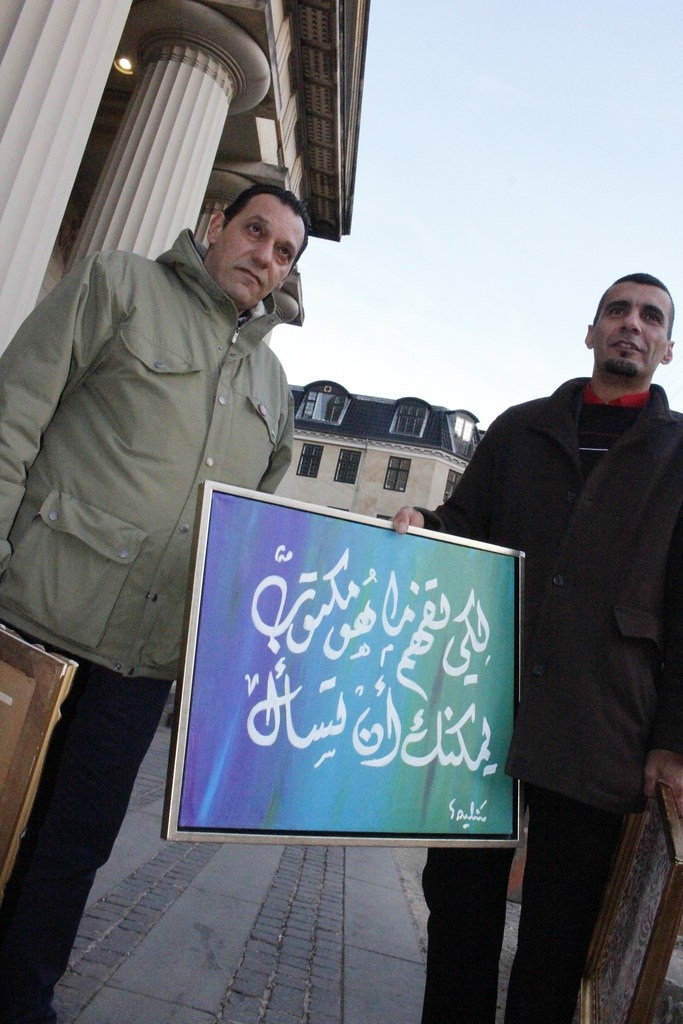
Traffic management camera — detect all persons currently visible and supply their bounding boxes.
[393,272,683,1024]
[0,183,311,1024]
